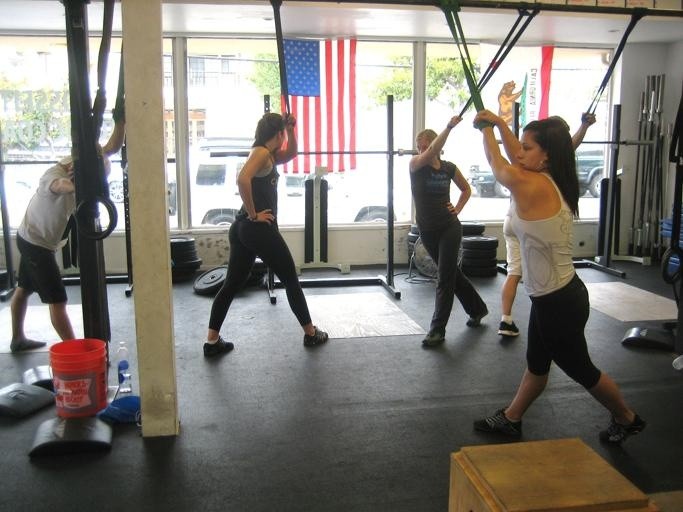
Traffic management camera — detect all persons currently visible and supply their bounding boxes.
[9,99,126,351]
[498,112,595,337]
[407,116,489,347]
[473,106,646,447]
[203,112,329,357]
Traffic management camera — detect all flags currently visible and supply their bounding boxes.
[277,37,357,177]
[479,42,553,173]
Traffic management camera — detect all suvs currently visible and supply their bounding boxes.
[466,131,607,197]
[187,131,400,229]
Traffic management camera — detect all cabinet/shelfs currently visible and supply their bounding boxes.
[448,438,659,512]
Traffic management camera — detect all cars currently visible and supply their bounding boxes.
[2,151,177,234]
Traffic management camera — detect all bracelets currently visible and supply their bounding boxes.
[478,120,492,130]
[447,123,453,129]
[250,212,258,220]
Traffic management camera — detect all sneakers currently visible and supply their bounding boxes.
[423,329,445,345]
[474,410,521,435]
[498,321,520,336]
[304,326,327,346]
[204,336,233,355]
[600,413,645,445]
[467,304,488,326]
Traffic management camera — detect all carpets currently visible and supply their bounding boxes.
[0,304,84,353]
[583,282,679,322]
[304,292,428,339]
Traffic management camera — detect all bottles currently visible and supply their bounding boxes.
[116,340,133,393]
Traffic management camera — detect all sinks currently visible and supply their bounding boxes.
[48,339,110,418]
